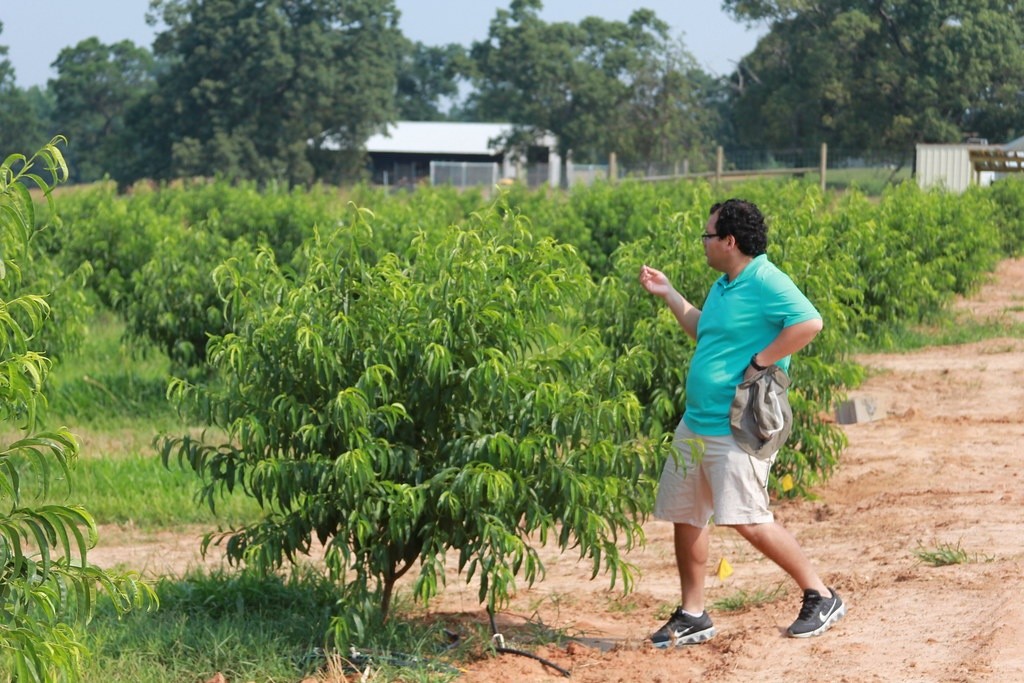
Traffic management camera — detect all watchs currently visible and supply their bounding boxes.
[751,353,767,371]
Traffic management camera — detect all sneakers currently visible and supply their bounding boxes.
[787,587,846,638]
[650,606,717,648]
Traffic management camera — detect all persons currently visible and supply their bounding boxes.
[640,198,846,648]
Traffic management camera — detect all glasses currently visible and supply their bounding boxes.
[701,231,718,243]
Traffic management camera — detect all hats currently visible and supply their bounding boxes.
[730,365,793,460]
